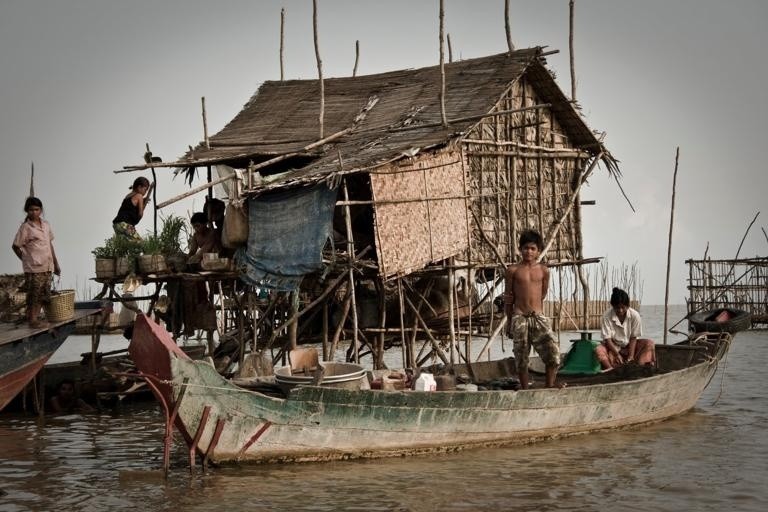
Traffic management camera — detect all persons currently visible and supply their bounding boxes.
[501,229,561,390]
[595,286,656,374]
[112,176,152,245]
[195,198,239,260]
[49,377,95,415]
[186,212,220,256]
[12,195,61,332]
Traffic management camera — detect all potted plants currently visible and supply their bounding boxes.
[91,209,193,278]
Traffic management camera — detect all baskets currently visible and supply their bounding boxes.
[41,289,75,322]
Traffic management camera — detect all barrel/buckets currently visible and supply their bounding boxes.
[277,363,366,392]
[414,369,437,392]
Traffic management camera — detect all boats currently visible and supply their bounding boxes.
[0,303,104,413]
[122,309,749,467]
